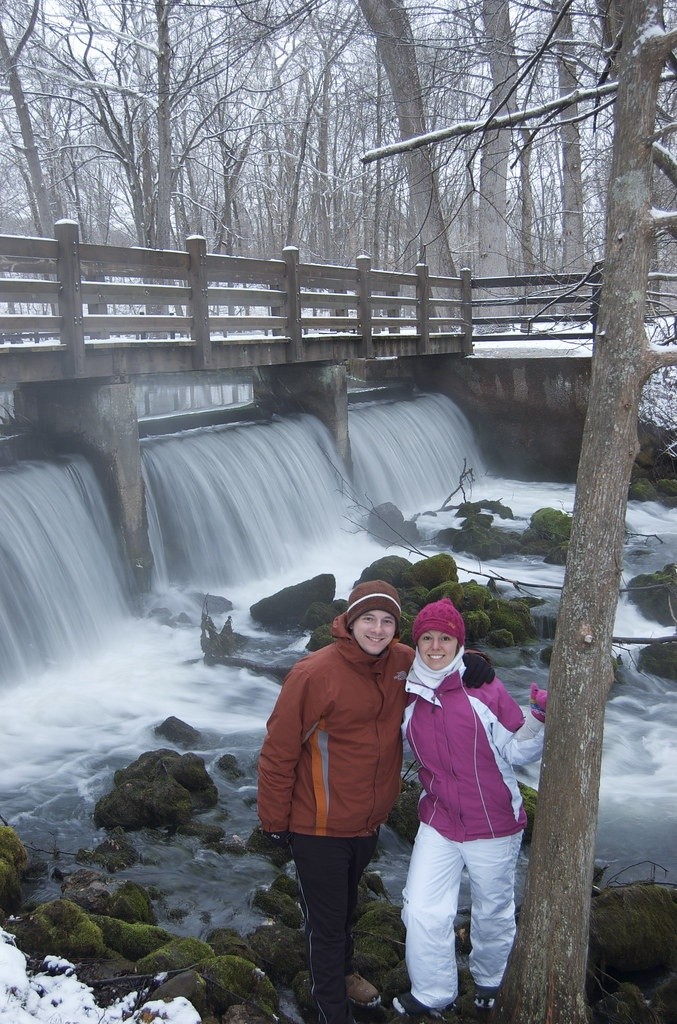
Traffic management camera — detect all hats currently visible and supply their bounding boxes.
[345,579,401,633]
[413,598,465,645]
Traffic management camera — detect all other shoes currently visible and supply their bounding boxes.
[391,993,453,1016]
[472,986,498,1010]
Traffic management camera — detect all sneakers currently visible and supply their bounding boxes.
[344,972,381,1007]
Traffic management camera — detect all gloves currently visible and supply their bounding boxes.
[463,654,495,689]
[267,831,290,845]
[529,683,551,723]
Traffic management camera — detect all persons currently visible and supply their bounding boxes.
[393,599,548,1015]
[258,579,496,1024]
